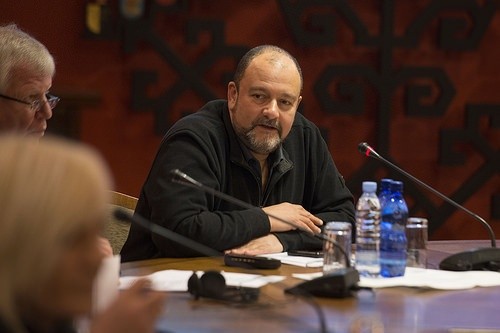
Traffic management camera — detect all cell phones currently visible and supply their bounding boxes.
[225,252,281,269]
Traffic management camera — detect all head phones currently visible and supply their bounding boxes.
[187,270,260,302]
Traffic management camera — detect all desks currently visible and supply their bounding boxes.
[88,240,500,333]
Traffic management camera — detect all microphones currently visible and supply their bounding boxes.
[113,206,328,333]
[357,141,500,271]
[168,169,359,297]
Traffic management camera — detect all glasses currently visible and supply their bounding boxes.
[0,91,61,113]
[188,269,261,305]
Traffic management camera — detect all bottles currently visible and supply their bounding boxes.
[353,181,382,278]
[379,181,409,278]
[377,178,394,212]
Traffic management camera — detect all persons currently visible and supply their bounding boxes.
[0,25,114,257]
[0,137,168,333]
[119,45,357,264]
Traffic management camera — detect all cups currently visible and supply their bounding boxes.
[323,222,352,273]
[406,217,429,271]
